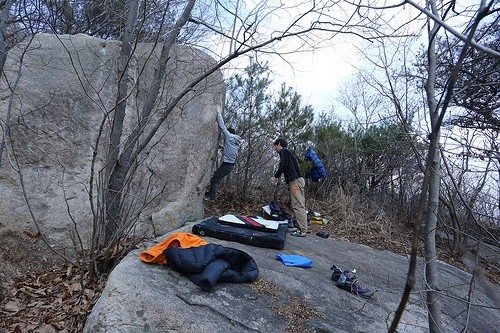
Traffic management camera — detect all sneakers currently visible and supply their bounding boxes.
[331,268,374,299]
[290,229,306,237]
[203,197,215,202]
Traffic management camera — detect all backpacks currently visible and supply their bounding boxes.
[262,201,287,221]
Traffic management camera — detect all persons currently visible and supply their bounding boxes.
[204,107,241,200]
[271,137,308,236]
